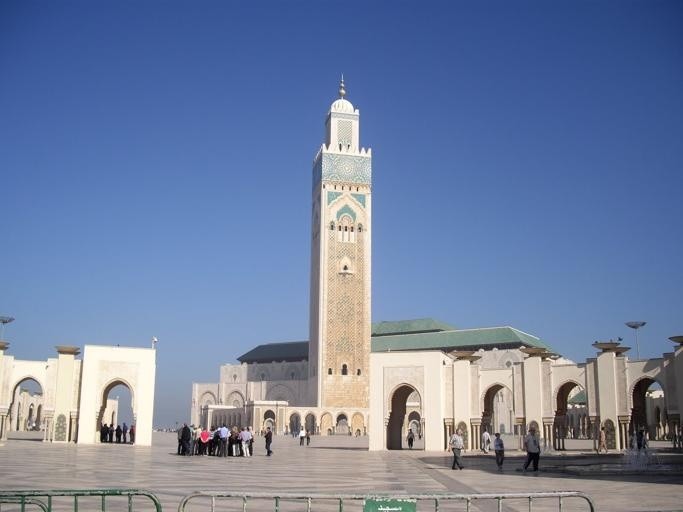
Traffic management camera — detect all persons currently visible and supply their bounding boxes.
[406,429,415,449]
[306,429,311,446]
[177,424,255,458]
[299,428,306,446]
[597,427,609,454]
[494,433,504,471]
[448,428,465,470]
[522,426,541,473]
[100,422,135,445]
[264,427,273,456]
[482,429,491,454]
